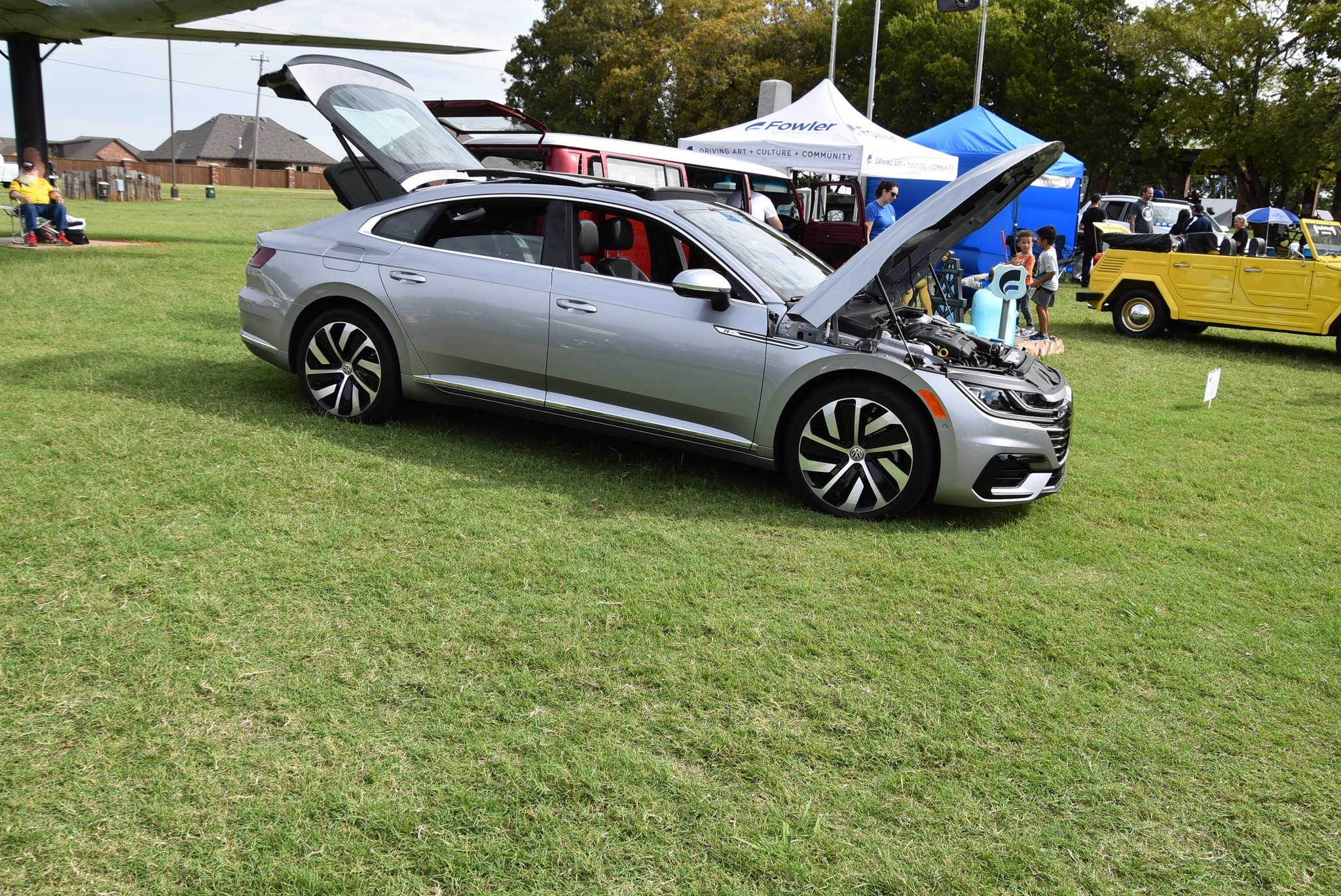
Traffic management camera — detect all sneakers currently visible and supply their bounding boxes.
[56,232,73,246]
[1020,326,1036,336]
[1030,333,1049,341]
[1029,331,1040,338]
[27,233,38,247]
[1015,325,1020,335]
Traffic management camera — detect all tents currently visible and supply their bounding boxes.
[677,78,959,339]
[864,105,1084,304]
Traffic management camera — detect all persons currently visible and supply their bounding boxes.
[1169,209,1190,236]
[865,179,899,244]
[1129,186,1154,234]
[1276,224,1296,247]
[1028,225,1059,340]
[48,159,59,192]
[9,161,73,247]
[1180,203,1213,235]
[1163,192,1169,198]
[1223,214,1248,255]
[1079,193,1108,288]
[725,178,783,232]
[1006,230,1036,336]
[1152,184,1164,198]
[1190,191,1199,204]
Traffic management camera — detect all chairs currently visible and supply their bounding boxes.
[1053,235,1082,282]
[596,217,650,281]
[1248,237,1267,257]
[1219,238,1237,255]
[577,219,601,274]
[0,196,62,244]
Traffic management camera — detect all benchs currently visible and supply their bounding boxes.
[415,211,540,264]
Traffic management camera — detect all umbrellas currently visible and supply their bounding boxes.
[1243,206,1300,254]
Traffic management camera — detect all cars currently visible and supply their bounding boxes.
[1077,194,1252,252]
[1076,218,1341,359]
[237,55,1074,520]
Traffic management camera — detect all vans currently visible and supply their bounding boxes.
[423,100,868,284]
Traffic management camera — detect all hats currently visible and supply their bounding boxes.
[20,161,35,169]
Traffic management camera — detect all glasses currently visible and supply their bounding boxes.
[886,190,898,198]
